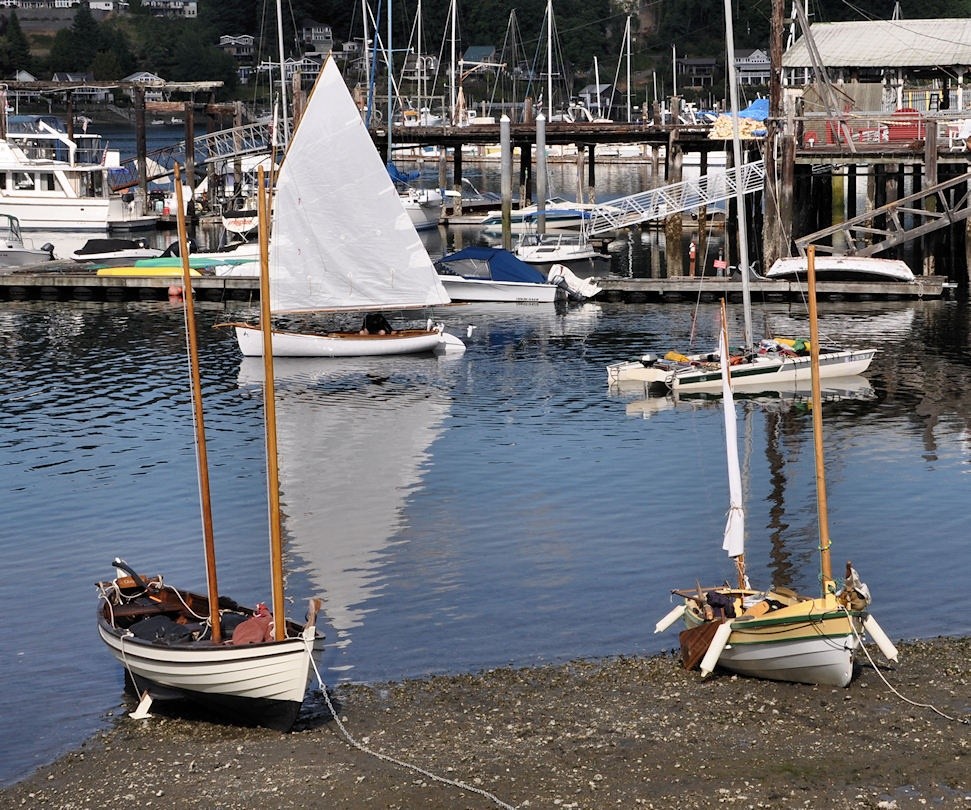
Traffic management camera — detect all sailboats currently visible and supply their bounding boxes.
[100,158,328,738]
[1,0,920,305]
[239,47,443,355]
[682,244,872,688]
[606,1,881,396]
